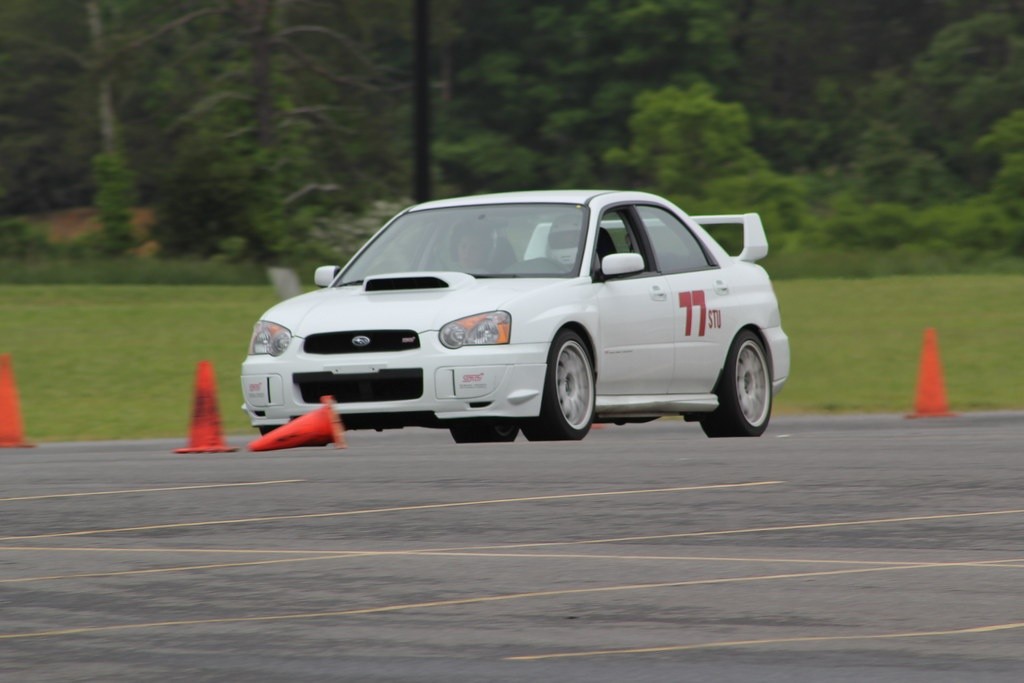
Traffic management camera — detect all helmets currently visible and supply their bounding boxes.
[548,210,599,266]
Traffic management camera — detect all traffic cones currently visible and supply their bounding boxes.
[0,354,36,448]
[250,394,349,450]
[170,360,240,455]
[901,326,956,418]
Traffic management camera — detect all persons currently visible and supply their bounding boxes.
[544,210,601,276]
[454,228,496,276]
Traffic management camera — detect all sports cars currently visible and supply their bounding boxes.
[238,187,794,436]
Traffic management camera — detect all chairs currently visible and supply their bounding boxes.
[596,229,620,279]
[483,236,517,272]
